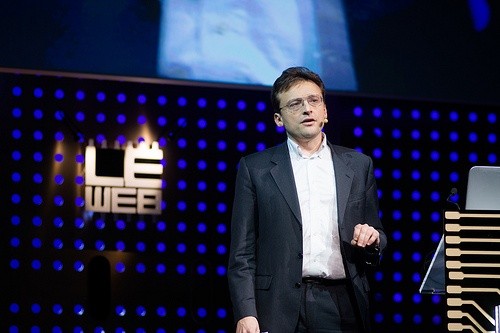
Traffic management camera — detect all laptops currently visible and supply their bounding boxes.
[464,165,500,211]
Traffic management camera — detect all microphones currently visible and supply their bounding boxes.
[447,188,460,210]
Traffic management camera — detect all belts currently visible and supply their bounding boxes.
[302,275,344,286]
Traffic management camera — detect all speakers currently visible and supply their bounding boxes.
[323,118,328,123]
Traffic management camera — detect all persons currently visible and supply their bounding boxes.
[228,66,387,333]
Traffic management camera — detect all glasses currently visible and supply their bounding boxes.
[277,95,324,114]
